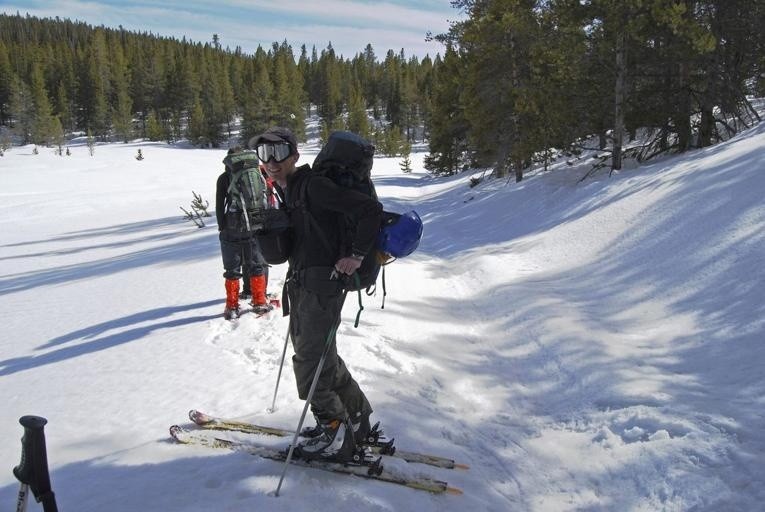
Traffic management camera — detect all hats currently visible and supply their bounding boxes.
[248,124,298,152]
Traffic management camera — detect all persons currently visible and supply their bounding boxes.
[241,164,276,299]
[216,145,273,318]
[248,127,383,460]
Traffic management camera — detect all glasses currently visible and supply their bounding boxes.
[255,143,296,165]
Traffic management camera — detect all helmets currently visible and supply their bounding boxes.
[376,209,423,259]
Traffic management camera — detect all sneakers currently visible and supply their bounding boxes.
[296,413,356,458]
[253,303,274,314]
[224,307,240,320]
[300,410,372,443]
[239,291,251,299]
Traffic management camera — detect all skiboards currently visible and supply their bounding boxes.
[169,410,469,494]
[224,297,274,321]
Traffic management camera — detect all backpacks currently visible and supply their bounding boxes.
[218,151,270,246]
[280,130,397,329]
